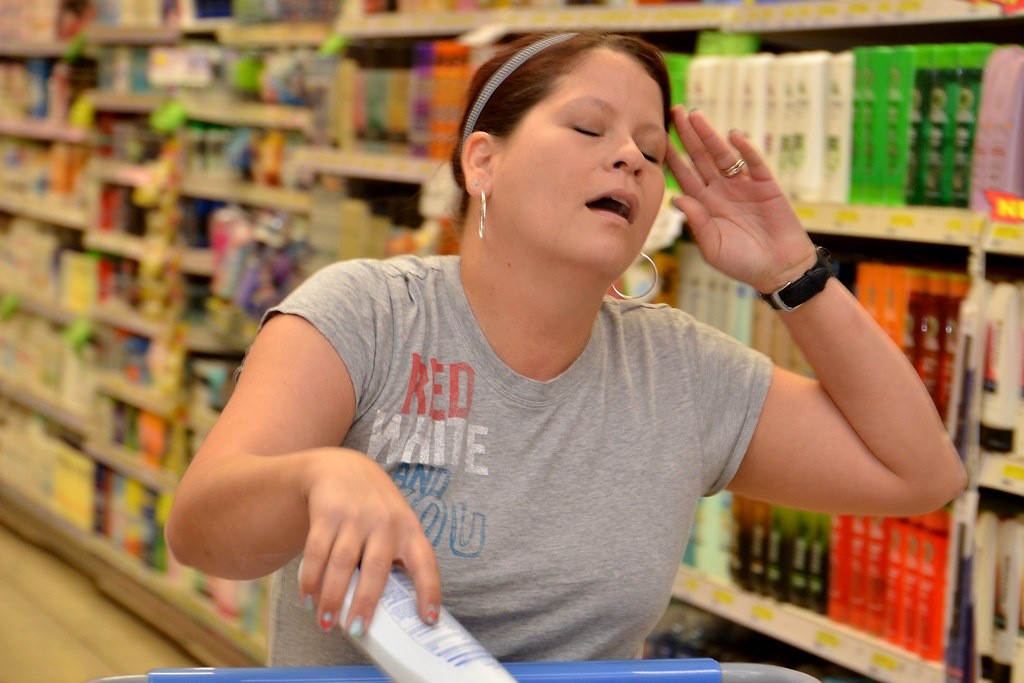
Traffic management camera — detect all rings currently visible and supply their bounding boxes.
[722,158,745,177]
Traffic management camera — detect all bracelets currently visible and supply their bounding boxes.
[758,243,839,312]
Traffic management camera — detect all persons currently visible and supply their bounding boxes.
[165,31,968,669]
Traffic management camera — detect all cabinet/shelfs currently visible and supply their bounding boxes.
[0,1,1024,683]
